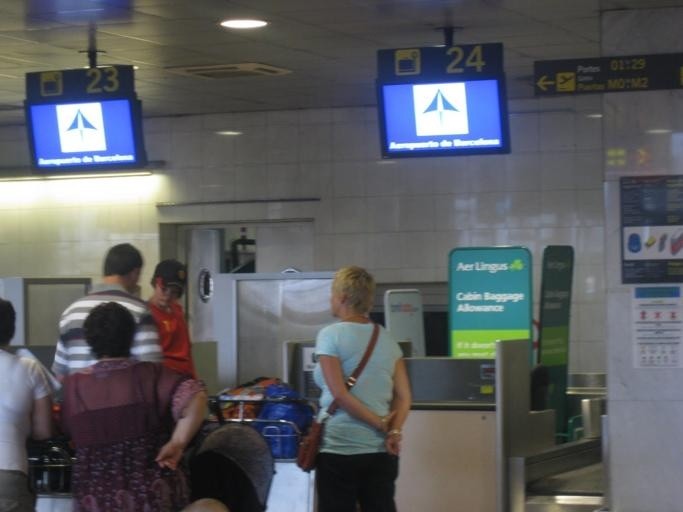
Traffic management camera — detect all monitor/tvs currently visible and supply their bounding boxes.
[372,71,512,158]
[22,93,148,173]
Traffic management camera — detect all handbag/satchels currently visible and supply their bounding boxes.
[208,375,312,459]
[294,412,330,472]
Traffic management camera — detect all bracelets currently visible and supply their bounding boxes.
[374,414,386,434]
[388,430,401,439]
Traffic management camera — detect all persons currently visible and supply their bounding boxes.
[50,241,165,393]
[60,301,210,512]
[307,263,413,511]
[144,258,197,381]
[0,297,55,512]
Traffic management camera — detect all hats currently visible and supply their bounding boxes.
[152,259,187,293]
